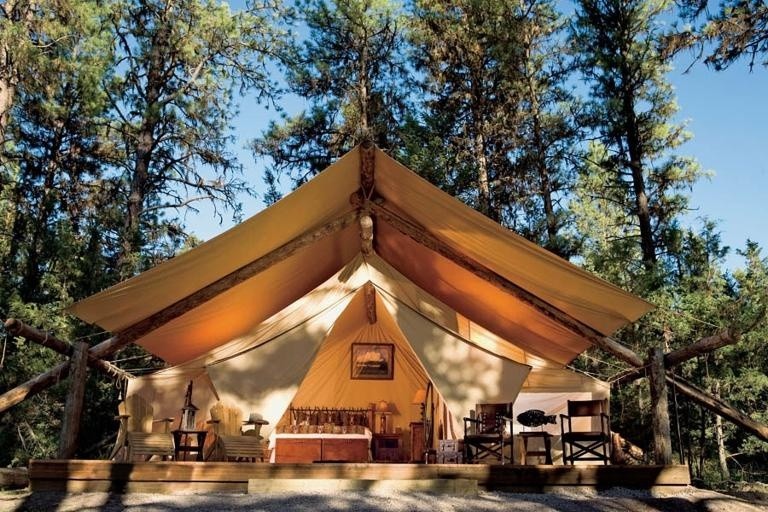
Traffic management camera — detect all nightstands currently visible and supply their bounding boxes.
[372,434,400,463]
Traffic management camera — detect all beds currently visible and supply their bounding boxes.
[275,403,373,464]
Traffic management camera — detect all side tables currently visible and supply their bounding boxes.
[517,431,553,464]
[171,429,208,461]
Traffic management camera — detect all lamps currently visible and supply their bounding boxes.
[411,389,428,420]
[375,400,393,435]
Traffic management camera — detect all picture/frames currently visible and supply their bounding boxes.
[351,343,395,381]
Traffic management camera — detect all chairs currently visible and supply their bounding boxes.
[461,402,514,466]
[204,401,269,462]
[559,398,615,466]
[107,393,174,463]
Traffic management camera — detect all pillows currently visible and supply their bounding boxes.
[281,422,366,434]
